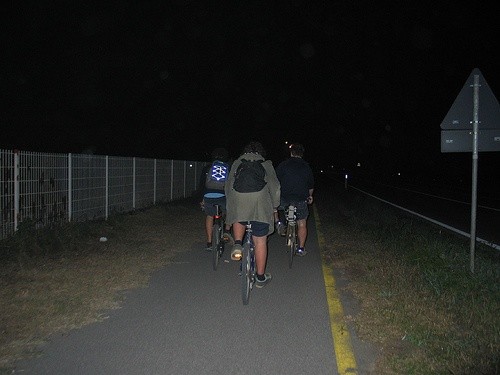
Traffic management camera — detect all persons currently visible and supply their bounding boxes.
[223,142,280,289]
[275,144,314,256]
[199,149,235,250]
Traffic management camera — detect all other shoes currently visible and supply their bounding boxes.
[231,244,243,260]
[255,272,271,288]
[224,230,230,238]
[205,243,212,248]
[278,224,285,236]
[295,249,307,256]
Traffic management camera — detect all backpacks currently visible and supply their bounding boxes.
[233,158,267,193]
[211,162,229,182]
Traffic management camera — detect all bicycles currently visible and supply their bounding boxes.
[201,199,229,269]
[279,203,305,268]
[236,220,264,305]
